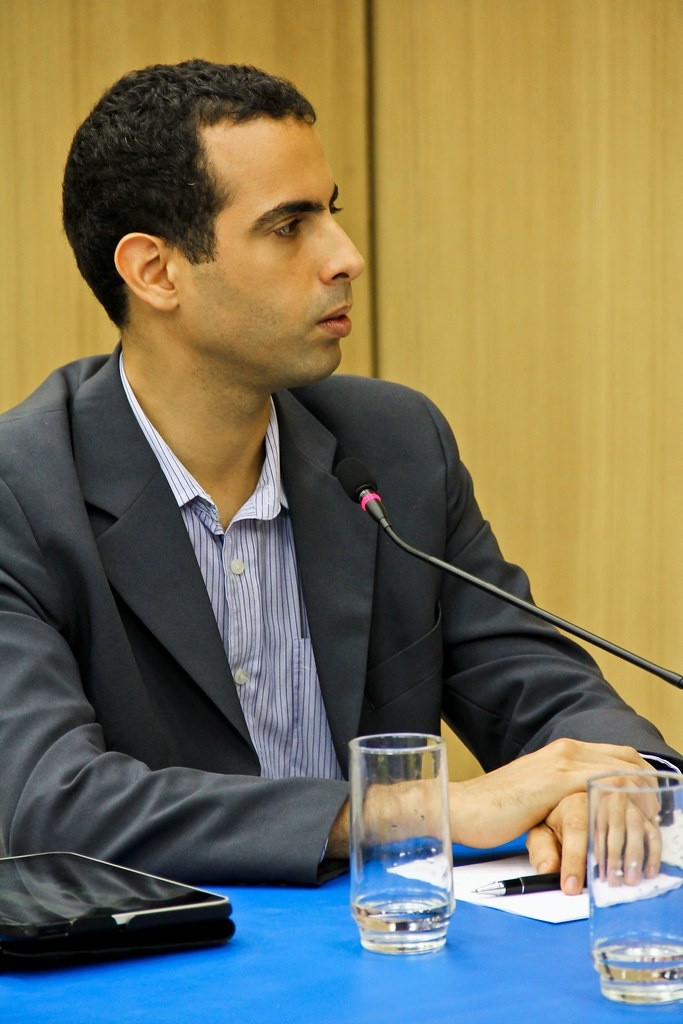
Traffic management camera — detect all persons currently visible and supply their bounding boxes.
[0,56,683,897]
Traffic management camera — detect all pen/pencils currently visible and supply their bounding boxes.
[470,871,588,896]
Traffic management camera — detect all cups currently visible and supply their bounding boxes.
[349,732,457,953]
[586,771,683,1007]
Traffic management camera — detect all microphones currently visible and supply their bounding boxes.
[336,458,683,691]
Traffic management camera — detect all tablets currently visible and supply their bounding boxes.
[0,849,229,943]
